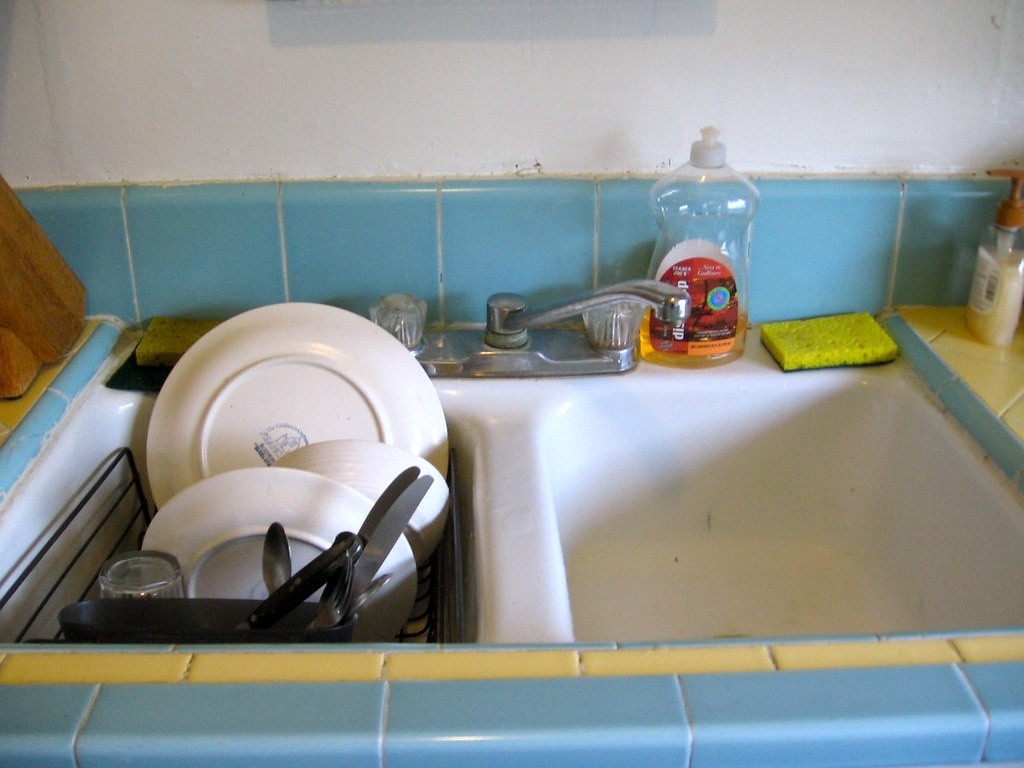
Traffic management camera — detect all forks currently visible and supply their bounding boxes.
[304,556,359,631]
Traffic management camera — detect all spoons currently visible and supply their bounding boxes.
[348,573,396,621]
[261,521,291,602]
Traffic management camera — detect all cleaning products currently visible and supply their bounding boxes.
[966,169,1024,348]
[634,126,762,370]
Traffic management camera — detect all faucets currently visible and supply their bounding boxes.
[486,278,691,352]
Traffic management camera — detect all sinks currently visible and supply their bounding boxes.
[0,384,525,649]
[525,362,1024,646]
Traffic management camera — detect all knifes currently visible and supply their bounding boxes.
[355,476,434,593]
[357,467,420,549]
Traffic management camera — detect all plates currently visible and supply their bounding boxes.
[146,302,451,497]
[270,439,450,562]
[142,465,416,639]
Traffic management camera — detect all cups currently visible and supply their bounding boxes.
[97,551,186,623]
